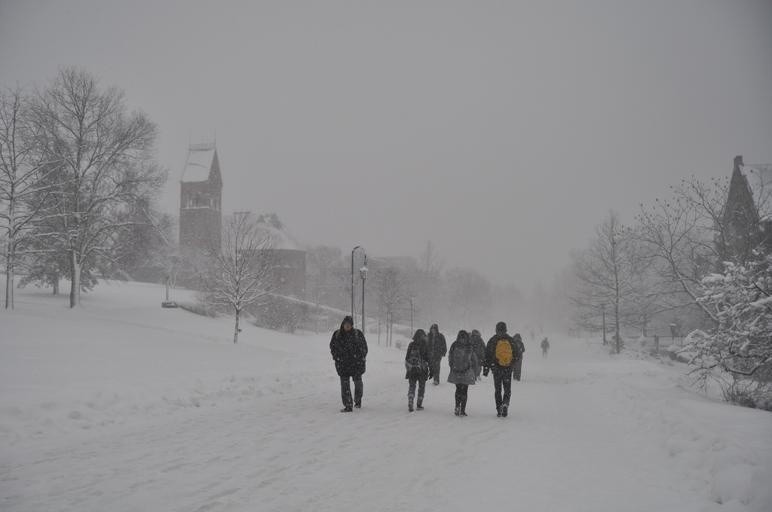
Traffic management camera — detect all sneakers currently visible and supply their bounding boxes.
[340,402,508,418]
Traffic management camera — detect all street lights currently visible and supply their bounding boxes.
[349,243,368,318]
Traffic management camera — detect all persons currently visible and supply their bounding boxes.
[448,330,479,416]
[511,333,525,382]
[471,329,486,381]
[485,322,519,418]
[330,317,367,413]
[405,329,435,412]
[541,337,550,359]
[426,325,447,385]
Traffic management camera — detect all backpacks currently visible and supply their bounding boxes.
[406,345,427,377]
[450,343,473,373]
[493,337,513,368]
